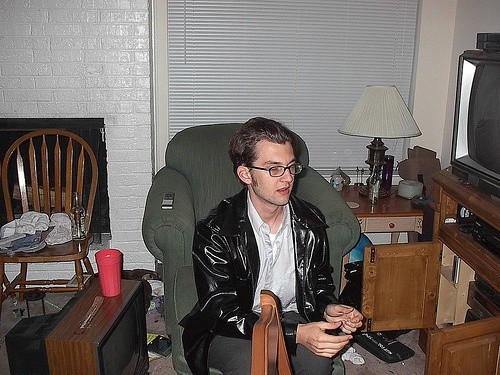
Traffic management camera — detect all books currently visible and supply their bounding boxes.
[147,333,172,362]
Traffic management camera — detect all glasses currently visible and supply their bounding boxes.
[242,162,303,177]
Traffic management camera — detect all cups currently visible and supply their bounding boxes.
[95,249,122,298]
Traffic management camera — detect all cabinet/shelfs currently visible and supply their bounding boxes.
[362,166,500,375]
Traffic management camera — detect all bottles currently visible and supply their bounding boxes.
[69,192,88,241]
[368,164,381,206]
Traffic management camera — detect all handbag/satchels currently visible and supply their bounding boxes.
[337,260,412,338]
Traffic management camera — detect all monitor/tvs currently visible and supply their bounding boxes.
[5,277,150,375]
[450,50,500,199]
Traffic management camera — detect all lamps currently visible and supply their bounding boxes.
[338,85,422,197]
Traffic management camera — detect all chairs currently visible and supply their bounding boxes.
[250,289,293,375]
[0,128,98,316]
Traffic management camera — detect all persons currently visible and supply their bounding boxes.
[176,117,365,375]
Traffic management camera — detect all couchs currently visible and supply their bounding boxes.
[144,122,361,375]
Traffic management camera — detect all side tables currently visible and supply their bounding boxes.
[338,185,423,295]
